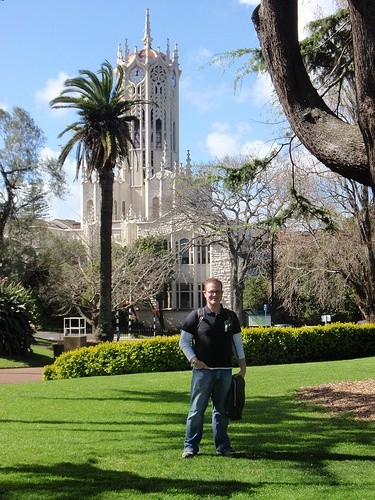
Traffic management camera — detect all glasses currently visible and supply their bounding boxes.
[205,289,223,295]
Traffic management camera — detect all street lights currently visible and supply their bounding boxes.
[268,233,277,327]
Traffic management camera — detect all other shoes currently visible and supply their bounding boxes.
[182,452,194,458]
[216,447,237,457]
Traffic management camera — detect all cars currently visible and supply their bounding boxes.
[274,323,295,328]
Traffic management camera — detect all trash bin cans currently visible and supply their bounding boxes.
[52,343,65,361]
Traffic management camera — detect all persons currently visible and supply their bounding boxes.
[179,278,246,460]
